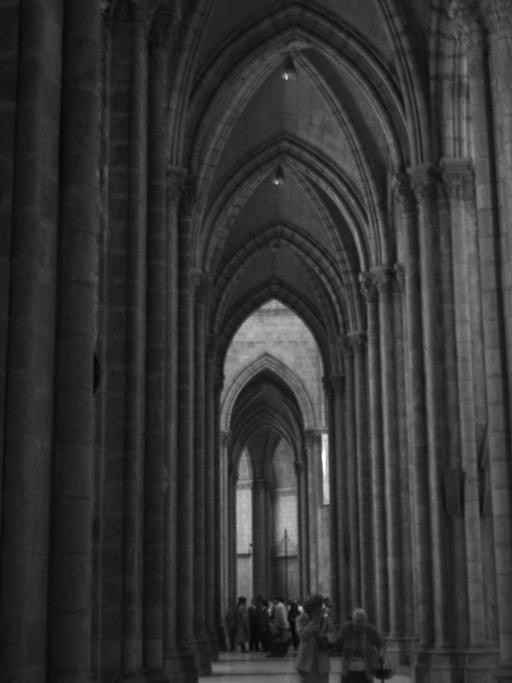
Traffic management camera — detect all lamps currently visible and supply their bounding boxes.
[272,164,286,185]
[279,55,299,82]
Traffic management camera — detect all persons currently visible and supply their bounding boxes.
[295,593,339,683]
[338,609,386,683]
[226,595,303,657]
